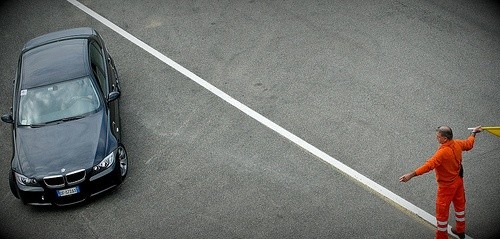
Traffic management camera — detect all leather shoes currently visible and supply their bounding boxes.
[451,226,465,239]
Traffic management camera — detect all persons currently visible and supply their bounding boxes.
[398,126,485,239]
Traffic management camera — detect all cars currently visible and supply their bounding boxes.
[0,26,128,208]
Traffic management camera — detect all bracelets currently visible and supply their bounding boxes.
[471,131,476,134]
